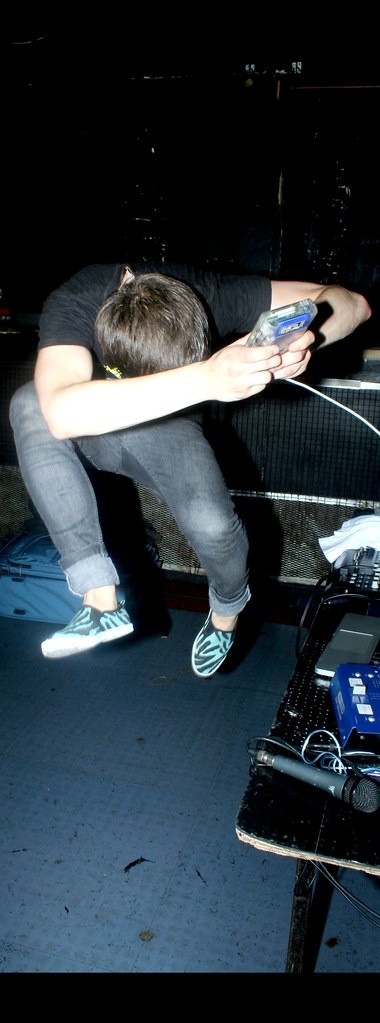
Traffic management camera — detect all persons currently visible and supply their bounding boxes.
[8,258,371,679]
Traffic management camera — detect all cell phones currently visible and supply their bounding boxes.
[315,612,380,678]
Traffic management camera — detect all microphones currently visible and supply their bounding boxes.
[247,747,379,814]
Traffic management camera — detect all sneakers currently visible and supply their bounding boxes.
[41,603,134,658]
[190,607,239,679]
